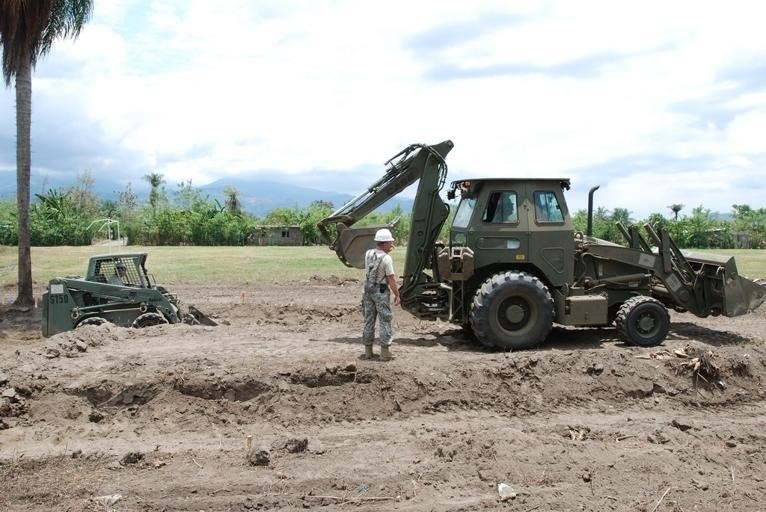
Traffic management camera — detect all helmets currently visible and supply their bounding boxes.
[114,263,126,269]
[374,228,395,242]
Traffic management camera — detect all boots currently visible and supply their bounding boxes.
[380,344,396,361]
[365,345,373,359]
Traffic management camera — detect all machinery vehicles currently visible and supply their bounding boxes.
[40,252,217,339]
[317,140,766,351]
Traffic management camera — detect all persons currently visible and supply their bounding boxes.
[491,195,513,224]
[362,228,400,361]
[109,263,127,286]
